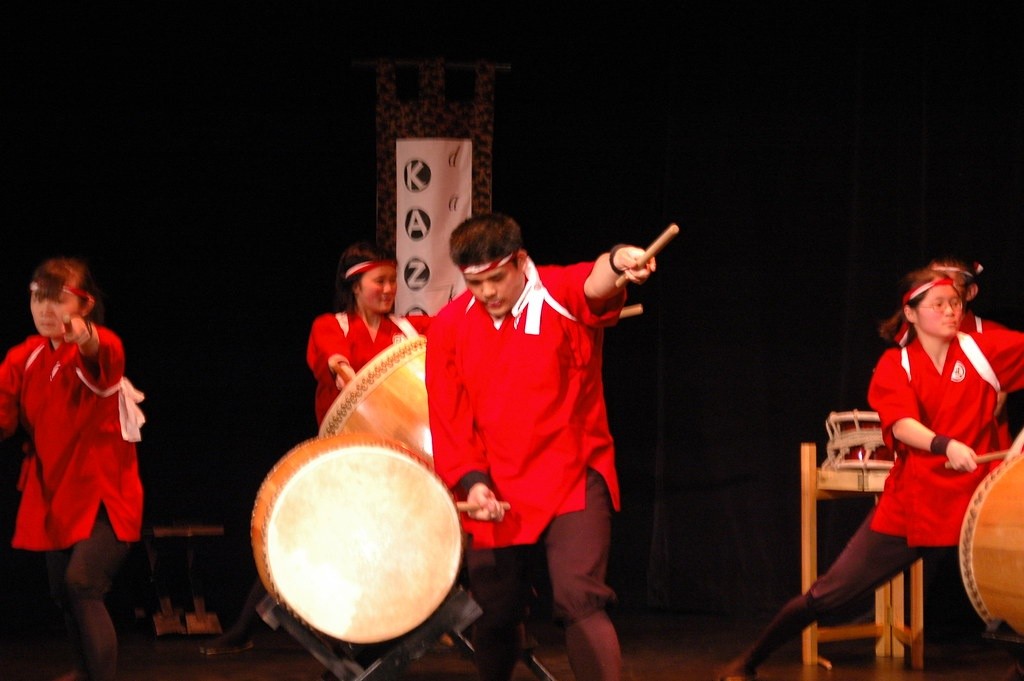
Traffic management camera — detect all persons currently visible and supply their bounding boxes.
[425,215,656,681]
[712,267,1010,680]
[305,248,404,439]
[878,252,1024,401]
[0,259,145,681]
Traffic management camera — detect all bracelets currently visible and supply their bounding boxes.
[609,243,633,276]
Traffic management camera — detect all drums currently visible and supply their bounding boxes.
[250,434,463,647]
[317,336,433,468]
[827,411,899,468]
[958,454,1024,635]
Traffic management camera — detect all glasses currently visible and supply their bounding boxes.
[919,297,963,312]
